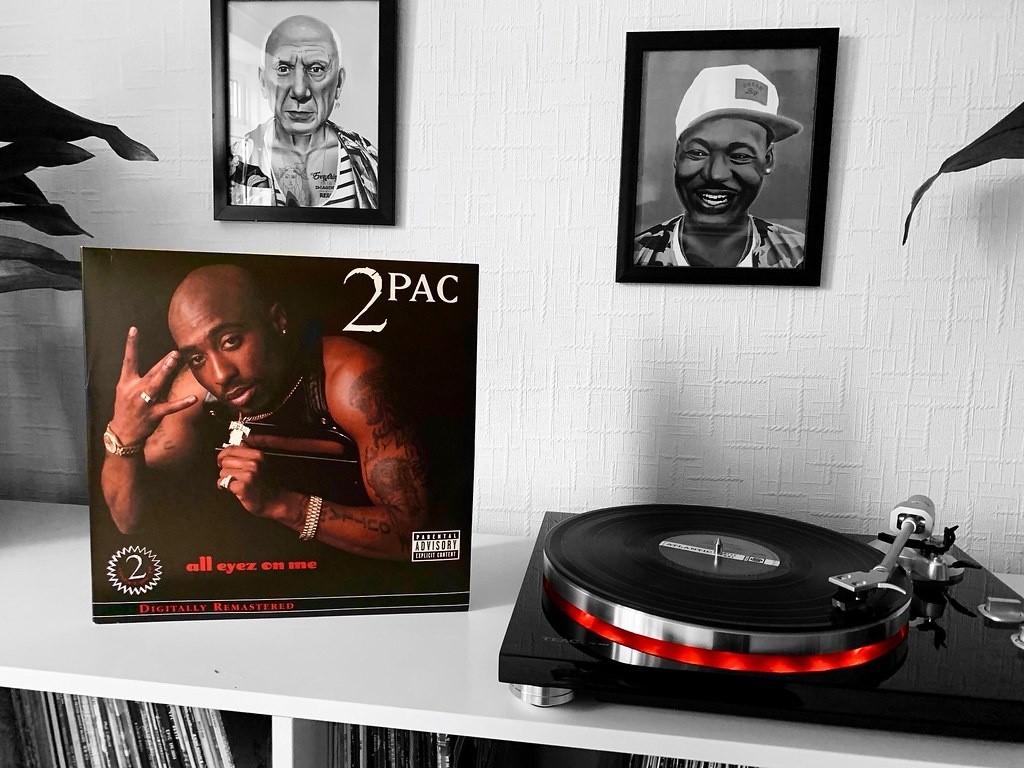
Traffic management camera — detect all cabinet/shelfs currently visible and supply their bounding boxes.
[0,498,1024,768]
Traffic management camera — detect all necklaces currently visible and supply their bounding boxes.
[208,377,304,422]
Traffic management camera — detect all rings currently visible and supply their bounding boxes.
[220,475,236,489]
[141,391,156,403]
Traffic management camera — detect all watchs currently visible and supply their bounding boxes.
[104,420,145,456]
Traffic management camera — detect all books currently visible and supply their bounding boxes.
[621,754,753,768]
[10,688,261,768]
[325,722,512,768]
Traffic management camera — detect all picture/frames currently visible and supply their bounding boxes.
[616,27,841,287]
[210,0,397,225]
[82,245,480,625]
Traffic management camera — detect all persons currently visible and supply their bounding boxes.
[101,265,429,563]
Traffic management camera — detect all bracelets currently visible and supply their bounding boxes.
[299,495,323,541]
